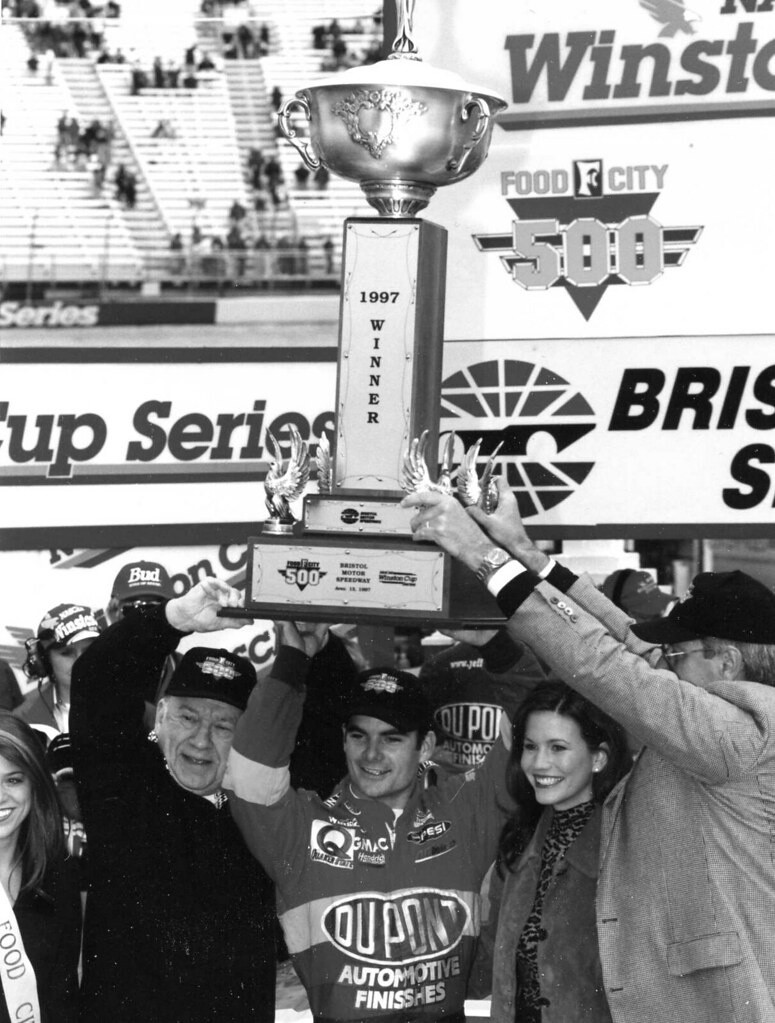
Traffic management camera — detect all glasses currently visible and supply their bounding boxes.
[661,646,716,667]
[114,604,160,617]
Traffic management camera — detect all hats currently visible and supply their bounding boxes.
[37,602,104,647]
[111,560,171,601]
[338,665,425,734]
[164,647,257,712]
[601,569,680,615]
[630,569,775,644]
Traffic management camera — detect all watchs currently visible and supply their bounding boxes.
[475,548,508,579]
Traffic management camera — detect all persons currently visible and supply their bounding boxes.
[12,602,102,856]
[220,620,547,1023]
[601,569,680,622]
[0,709,84,1022]
[0,0,382,277]
[70,576,359,1023]
[108,562,176,622]
[400,477,774,1023]
[419,642,547,679]
[466,679,635,1023]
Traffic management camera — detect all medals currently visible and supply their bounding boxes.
[217,1,508,630]
